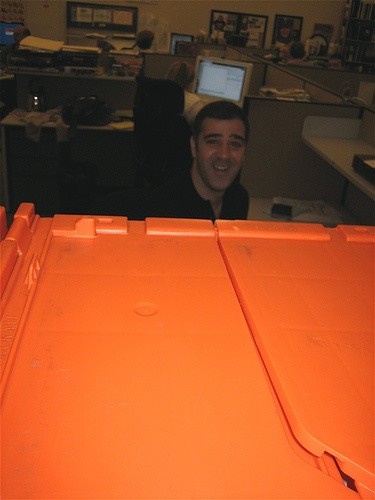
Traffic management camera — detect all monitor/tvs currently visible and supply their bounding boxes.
[192,55,254,110]
[0,21,24,47]
[169,32,193,57]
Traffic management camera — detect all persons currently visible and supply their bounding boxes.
[167,60,203,120]
[136,30,155,49]
[212,15,226,33]
[275,18,296,44]
[149,100,250,220]
[328,54,346,71]
[287,41,308,66]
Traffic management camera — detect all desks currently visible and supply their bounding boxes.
[0,108,133,212]
[306,136,375,200]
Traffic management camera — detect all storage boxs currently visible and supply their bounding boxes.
[0,202,375,499]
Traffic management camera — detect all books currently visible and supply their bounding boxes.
[341,0,375,74]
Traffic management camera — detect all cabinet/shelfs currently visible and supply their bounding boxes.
[342,15,375,74]
